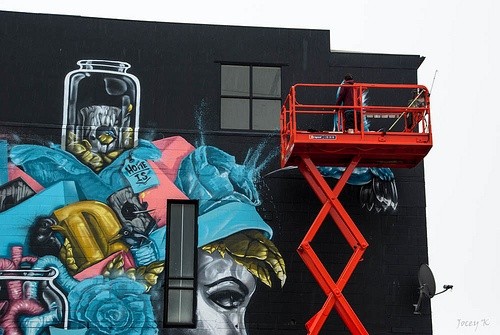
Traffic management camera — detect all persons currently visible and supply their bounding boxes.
[333,74,363,134]
